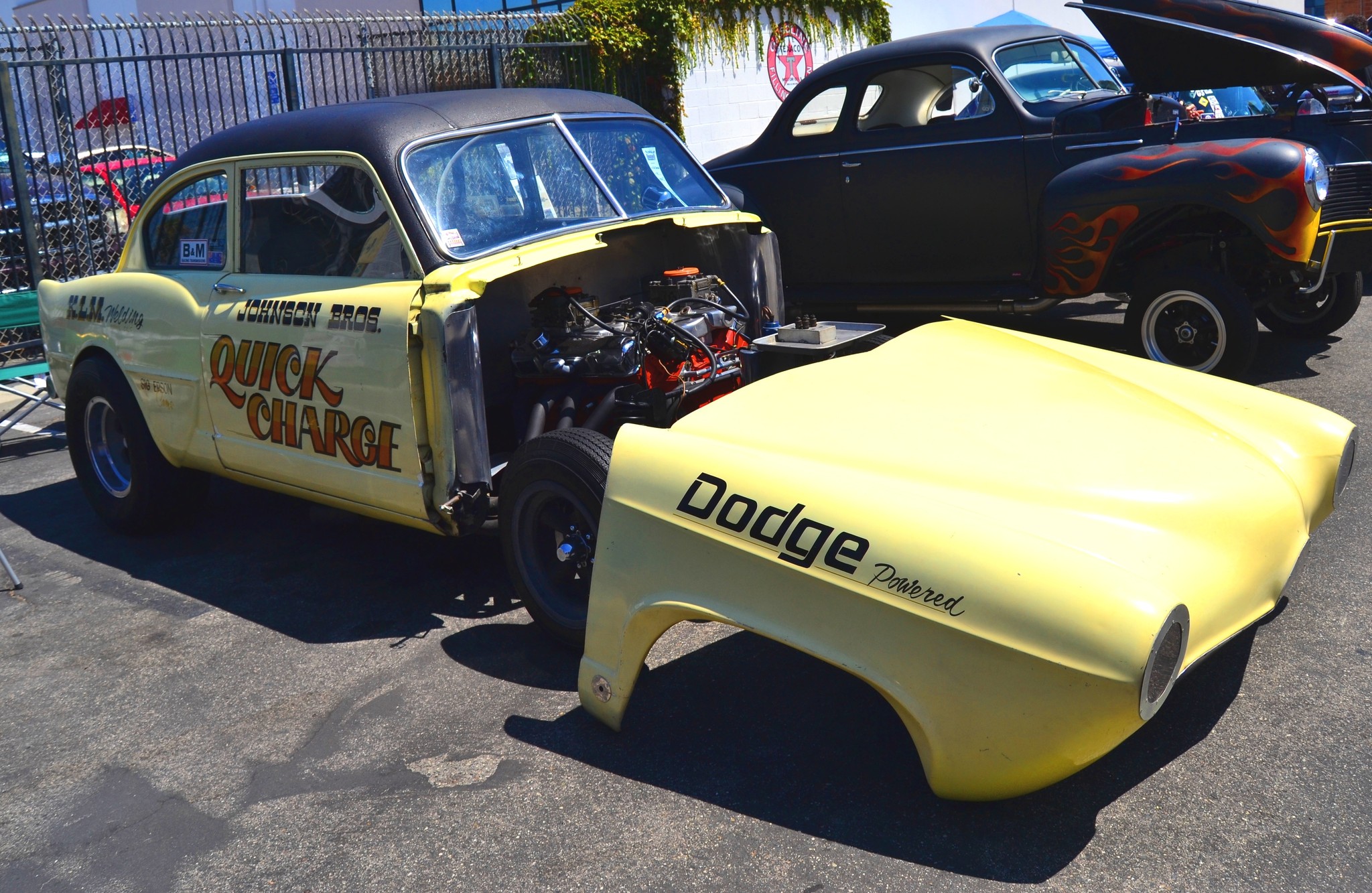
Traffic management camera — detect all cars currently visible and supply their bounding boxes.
[52,90,1359,802]
[1,144,269,294]
[670,0,1372,377]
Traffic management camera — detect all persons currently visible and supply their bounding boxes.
[1342,15,1372,89]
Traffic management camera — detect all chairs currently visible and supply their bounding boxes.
[0,290,66,433]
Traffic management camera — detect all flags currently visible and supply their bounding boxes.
[73,97,129,129]
